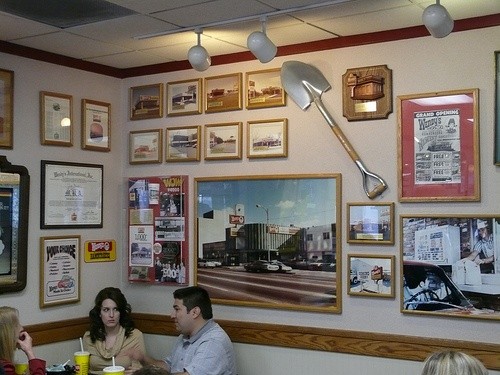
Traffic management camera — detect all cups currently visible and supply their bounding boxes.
[13,363,29,375]
[74,351,90,375]
[103,366,125,375]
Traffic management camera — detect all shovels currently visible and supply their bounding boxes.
[280,61,388,200]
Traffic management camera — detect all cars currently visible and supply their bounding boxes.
[271,260,293,273]
[198,259,221,268]
[403,261,478,314]
[244,260,280,272]
[282,258,336,272]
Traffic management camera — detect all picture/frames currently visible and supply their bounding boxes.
[166,125,201,162]
[194,174,343,315]
[245,67,286,109]
[347,253,395,298]
[0,68,14,150]
[399,213,500,320]
[81,98,111,153]
[397,88,480,203]
[166,78,202,117]
[347,202,393,245]
[203,72,243,114]
[129,128,163,165]
[247,118,288,158]
[129,83,163,121]
[204,122,243,161]
[0,155,30,294]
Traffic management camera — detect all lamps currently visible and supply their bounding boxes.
[246,16,278,64]
[422,0,454,38]
[187,28,212,72]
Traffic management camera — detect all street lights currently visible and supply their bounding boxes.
[256,204,270,261]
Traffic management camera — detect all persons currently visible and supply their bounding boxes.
[128,286,238,375]
[421,351,489,375]
[0,306,46,375]
[403,266,452,310]
[465,218,495,273]
[82,287,144,375]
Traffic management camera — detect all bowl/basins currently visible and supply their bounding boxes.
[46,364,73,375]
